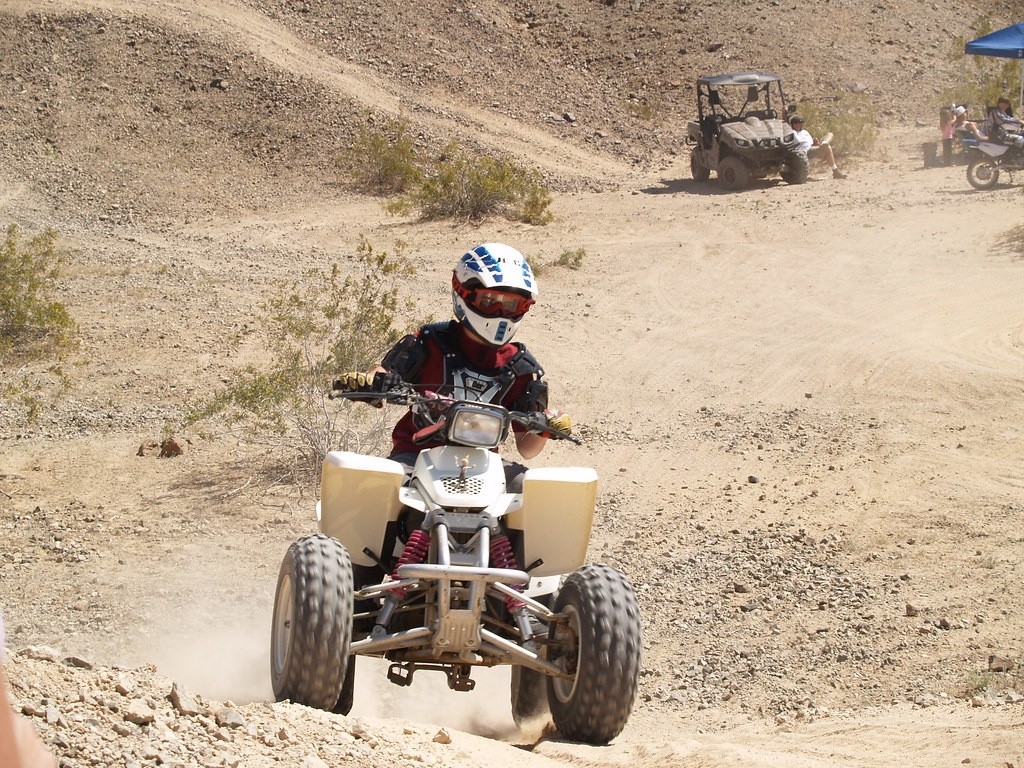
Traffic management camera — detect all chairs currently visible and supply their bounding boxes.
[953,130,980,161]
[705,114,726,146]
[746,111,765,121]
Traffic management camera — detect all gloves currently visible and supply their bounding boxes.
[535,406,573,441]
[338,369,384,409]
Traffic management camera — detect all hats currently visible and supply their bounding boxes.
[954,105,968,119]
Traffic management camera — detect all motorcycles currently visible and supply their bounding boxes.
[962,135,1023,187]
[268,374,643,749]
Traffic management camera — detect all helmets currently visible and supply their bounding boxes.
[452,242,540,350]
[791,116,805,124]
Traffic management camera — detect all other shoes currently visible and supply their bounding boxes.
[820,141,829,150]
[833,167,847,179]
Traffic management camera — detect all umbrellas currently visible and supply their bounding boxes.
[965,23,1024,123]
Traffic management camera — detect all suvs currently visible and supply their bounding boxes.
[684,71,813,188]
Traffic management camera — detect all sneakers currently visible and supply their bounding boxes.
[512,610,549,640]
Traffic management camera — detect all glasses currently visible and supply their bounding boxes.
[464,285,537,323]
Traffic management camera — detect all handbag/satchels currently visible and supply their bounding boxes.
[989,110,1007,145]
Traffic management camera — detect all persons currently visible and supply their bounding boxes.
[331,242,573,496]
[791,116,847,178]
[940,97,1024,167]
